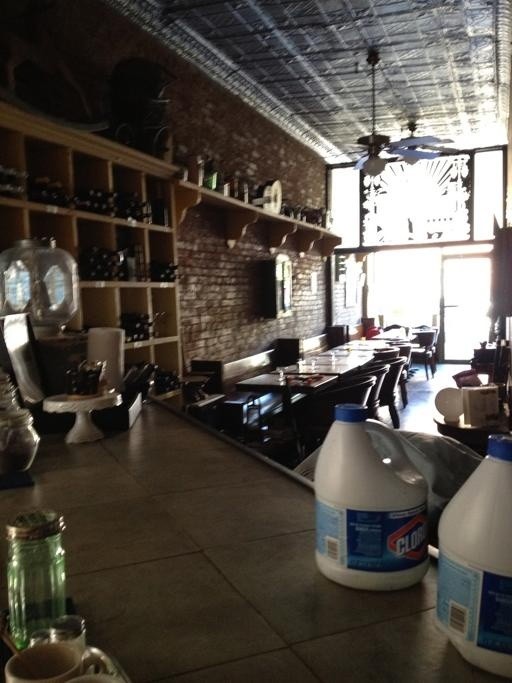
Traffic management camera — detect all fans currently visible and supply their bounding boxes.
[394,122,458,161]
[331,44,441,170]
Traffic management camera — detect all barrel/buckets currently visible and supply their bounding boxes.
[436,435,511,677]
[315,404,430,590]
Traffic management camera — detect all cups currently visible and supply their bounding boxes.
[4,644,107,683]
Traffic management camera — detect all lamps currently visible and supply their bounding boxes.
[363,146,386,177]
[405,148,421,164]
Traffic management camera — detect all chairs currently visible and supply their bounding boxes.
[298,324,438,458]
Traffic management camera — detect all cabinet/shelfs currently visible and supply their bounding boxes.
[1,100,184,414]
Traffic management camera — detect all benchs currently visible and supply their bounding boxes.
[190,337,303,425]
[301,326,347,361]
[347,318,375,342]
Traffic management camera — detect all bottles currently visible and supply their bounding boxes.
[0,163,181,395]
[175,143,323,227]
[0,372,41,474]
[27,629,50,649]
[49,614,86,658]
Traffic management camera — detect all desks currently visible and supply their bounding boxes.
[432,413,502,453]
[471,343,509,383]
